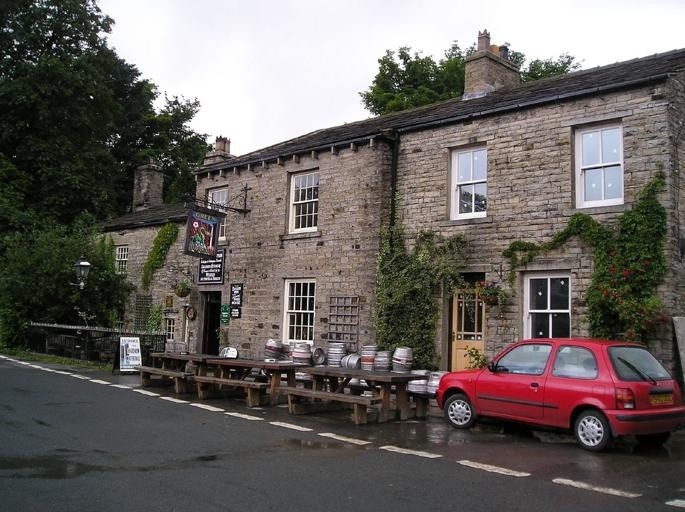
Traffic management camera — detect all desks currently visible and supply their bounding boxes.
[303,367,429,419]
[150,351,228,393]
[207,358,312,405]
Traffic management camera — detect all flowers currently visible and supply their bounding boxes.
[474,278,509,320]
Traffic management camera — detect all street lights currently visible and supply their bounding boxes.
[73,254,91,359]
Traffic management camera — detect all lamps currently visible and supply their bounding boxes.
[70,253,90,291]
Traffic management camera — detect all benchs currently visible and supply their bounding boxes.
[247,373,313,403]
[329,379,436,419]
[134,366,195,393]
[188,376,271,406]
[278,386,383,424]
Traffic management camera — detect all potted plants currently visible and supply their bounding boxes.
[172,277,193,298]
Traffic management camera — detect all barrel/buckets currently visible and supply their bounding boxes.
[264,335,452,410]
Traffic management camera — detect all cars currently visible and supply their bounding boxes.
[435,336,685,452]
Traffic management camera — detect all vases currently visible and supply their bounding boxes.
[480,294,498,306]
